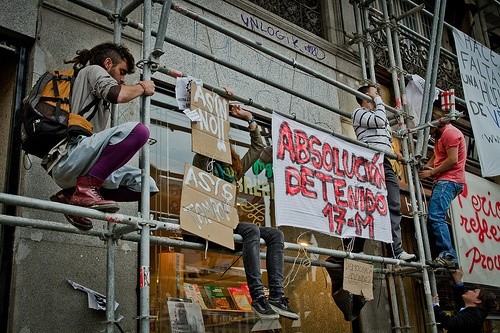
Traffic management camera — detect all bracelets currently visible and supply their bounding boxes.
[248,117,254,123]
[429,170,433,176]
[138,83,146,97]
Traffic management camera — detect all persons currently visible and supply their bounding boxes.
[351,85,417,263]
[418,110,467,266]
[193,87,299,320]
[432,267,496,333]
[41,42,159,229]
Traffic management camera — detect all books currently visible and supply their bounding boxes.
[184,283,252,310]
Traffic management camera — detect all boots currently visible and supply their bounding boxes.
[48,188,94,231]
[68,173,121,213]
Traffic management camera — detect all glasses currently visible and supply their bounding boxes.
[373,91,380,97]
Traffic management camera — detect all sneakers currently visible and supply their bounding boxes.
[250,294,280,322]
[425,253,460,270]
[268,289,300,321]
[390,248,417,262]
[352,250,375,265]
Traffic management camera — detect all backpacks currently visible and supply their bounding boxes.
[12,66,95,171]
